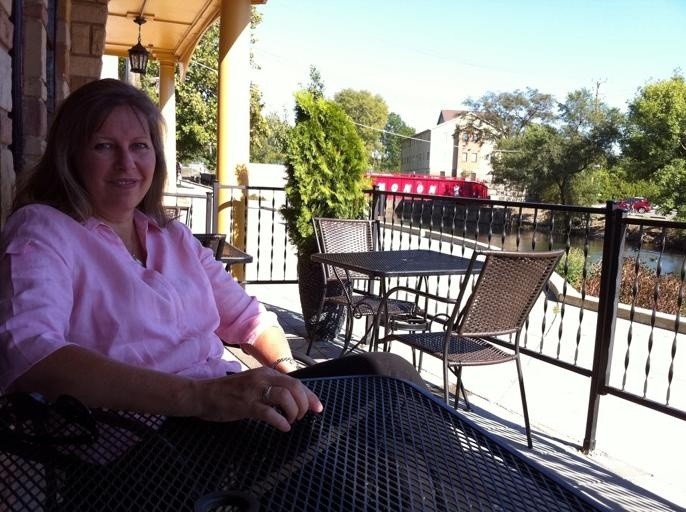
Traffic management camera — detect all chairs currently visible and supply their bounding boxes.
[310,215,425,360]
[162,205,191,228]
[192,234,226,260]
[386,248,565,449]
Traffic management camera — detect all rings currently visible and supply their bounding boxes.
[262,383,273,399]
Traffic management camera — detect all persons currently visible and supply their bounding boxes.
[0,79,433,434]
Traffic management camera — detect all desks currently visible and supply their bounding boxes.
[192,236,255,276]
[0,374,611,510]
[309,249,485,364]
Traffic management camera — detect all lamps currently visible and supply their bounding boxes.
[126,17,149,73]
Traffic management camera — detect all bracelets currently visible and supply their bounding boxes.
[272,357,296,372]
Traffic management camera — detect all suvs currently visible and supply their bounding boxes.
[620,197,651,214]
